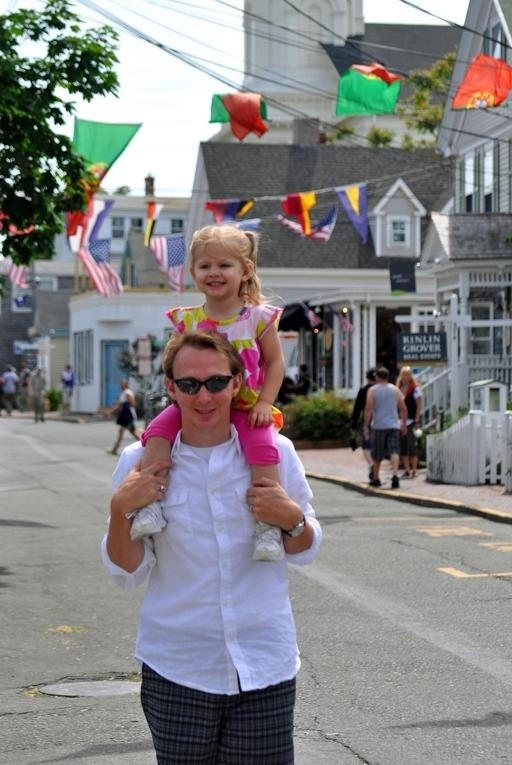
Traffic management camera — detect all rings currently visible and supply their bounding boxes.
[159,484,165,492]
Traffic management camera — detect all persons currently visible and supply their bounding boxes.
[1,362,76,422]
[295,363,310,398]
[130,225,287,564]
[99,378,142,455]
[348,367,376,481]
[360,369,408,488]
[393,367,422,478]
[277,374,295,407]
[100,329,325,765]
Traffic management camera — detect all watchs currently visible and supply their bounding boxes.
[280,513,307,537]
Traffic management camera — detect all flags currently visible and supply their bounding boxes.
[72,117,141,197]
[451,55,512,110]
[336,62,403,117]
[212,93,270,139]
[2,198,263,298]
[280,184,368,243]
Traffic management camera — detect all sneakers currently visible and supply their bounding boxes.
[251,520,286,561]
[125,501,167,542]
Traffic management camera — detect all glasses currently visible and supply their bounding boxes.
[169,370,236,395]
